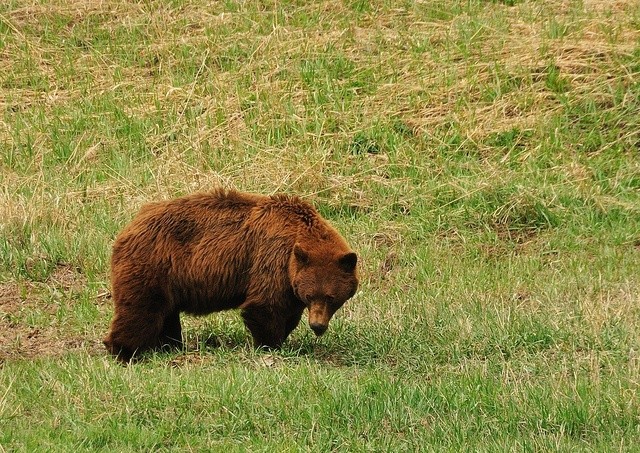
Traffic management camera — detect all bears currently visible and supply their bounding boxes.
[103,185,360,365]
